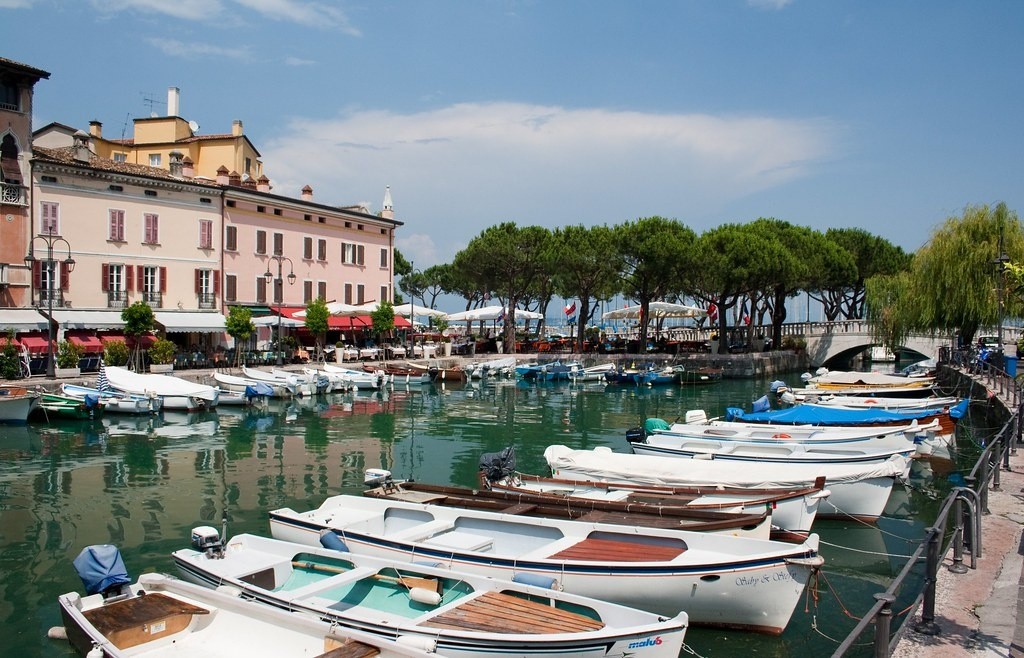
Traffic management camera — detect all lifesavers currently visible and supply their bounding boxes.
[772,434,793,439]
[865,399,878,405]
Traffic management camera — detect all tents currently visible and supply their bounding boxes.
[441,305,544,321]
[602,301,709,342]
[292,303,446,317]
[405,317,425,326]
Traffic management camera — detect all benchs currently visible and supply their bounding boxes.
[80,593,217,650]
[387,522,455,541]
[628,492,700,501]
[214,554,294,589]
[789,446,807,459]
[714,446,737,454]
[286,567,379,599]
[519,534,587,561]
[314,641,381,658]
[328,509,386,538]
[731,432,753,437]
[688,494,716,505]
[499,504,537,515]
[602,491,633,502]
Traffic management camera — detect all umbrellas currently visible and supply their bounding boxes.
[248,315,307,327]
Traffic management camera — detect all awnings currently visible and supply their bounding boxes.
[270,305,413,332]
[0,309,229,334]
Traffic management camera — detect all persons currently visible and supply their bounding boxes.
[466,334,475,346]
[758,333,765,339]
[614,333,622,348]
[651,336,656,344]
[356,334,380,349]
[660,336,667,343]
[201,336,206,346]
[392,336,401,348]
[497,335,503,341]
[172,343,202,369]
[530,339,534,344]
[451,336,459,343]
[484,327,491,338]
[206,344,225,358]
[589,336,602,352]
[734,329,740,342]
[523,328,530,343]
[552,337,555,341]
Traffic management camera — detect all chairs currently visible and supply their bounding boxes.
[175,351,287,370]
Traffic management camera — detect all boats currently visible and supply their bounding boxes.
[477,354,973,526]
[172,517,689,658]
[266,493,824,637]
[0,339,475,429]
[362,467,774,542]
[48,542,450,658]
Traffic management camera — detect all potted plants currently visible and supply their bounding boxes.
[443,337,452,357]
[495,337,503,354]
[758,334,765,351]
[55,340,87,379]
[468,335,477,354]
[711,335,719,354]
[146,337,178,373]
[334,342,345,364]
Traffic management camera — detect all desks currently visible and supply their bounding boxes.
[514,338,704,354]
[306,345,436,363]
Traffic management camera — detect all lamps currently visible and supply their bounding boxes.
[178,302,184,306]
[65,301,71,305]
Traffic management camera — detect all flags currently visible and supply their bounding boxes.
[494,306,507,322]
[564,302,577,324]
[740,302,750,326]
[706,301,718,326]
